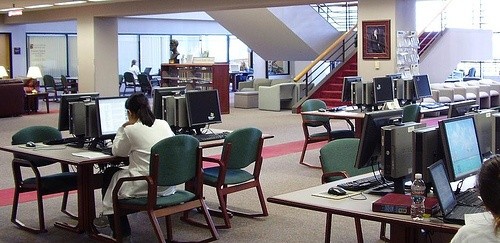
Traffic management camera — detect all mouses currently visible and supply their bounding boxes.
[328,186,346,196]
[318,109,325,112]
[26,141,35,147]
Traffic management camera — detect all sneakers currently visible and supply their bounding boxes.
[93,212,110,227]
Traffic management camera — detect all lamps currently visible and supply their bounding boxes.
[0,66,8,79]
[26,66,42,93]
[7,4,25,17]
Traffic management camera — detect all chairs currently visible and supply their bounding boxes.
[318,137,387,243]
[138,75,159,95]
[124,72,141,95]
[43,74,58,101]
[465,67,476,78]
[119,74,123,96]
[10,125,78,235]
[299,98,354,164]
[61,75,76,94]
[180,128,274,229]
[112,134,219,243]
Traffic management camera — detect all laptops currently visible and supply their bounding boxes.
[426,159,486,225]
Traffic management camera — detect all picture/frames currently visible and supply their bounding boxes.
[362,20,391,60]
[267,60,290,76]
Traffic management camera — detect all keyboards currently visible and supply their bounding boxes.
[337,175,389,192]
[455,185,483,207]
[193,133,225,142]
[43,137,75,145]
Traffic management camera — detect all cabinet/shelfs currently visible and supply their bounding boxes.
[161,62,230,114]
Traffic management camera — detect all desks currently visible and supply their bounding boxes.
[0,134,274,243]
[65,77,78,93]
[301,102,449,138]
[142,74,160,94]
[229,72,243,91]
[53,76,78,94]
[267,171,465,243]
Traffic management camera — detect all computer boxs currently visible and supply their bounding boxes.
[361,82,374,105]
[162,95,174,127]
[83,103,98,138]
[392,79,403,100]
[380,121,426,182]
[465,109,498,154]
[403,80,415,100]
[173,97,186,127]
[411,126,444,188]
[68,101,95,134]
[350,82,362,105]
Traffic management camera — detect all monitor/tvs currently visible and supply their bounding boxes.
[437,114,484,181]
[341,76,362,103]
[144,67,152,75]
[373,77,394,111]
[386,73,402,79]
[89,96,129,151]
[447,99,476,118]
[152,86,186,119]
[186,88,222,135]
[57,93,99,131]
[354,109,412,196]
[411,74,432,104]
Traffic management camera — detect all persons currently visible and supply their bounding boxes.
[92,93,177,241]
[370,28,383,51]
[169,38,180,59]
[450,153,500,243]
[129,58,142,79]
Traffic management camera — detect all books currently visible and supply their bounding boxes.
[371,192,442,218]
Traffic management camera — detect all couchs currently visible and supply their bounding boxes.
[0,79,23,118]
[258,83,297,111]
[293,83,315,101]
[238,79,294,91]
[23,79,39,110]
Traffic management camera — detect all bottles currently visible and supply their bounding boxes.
[410,172,426,221]
